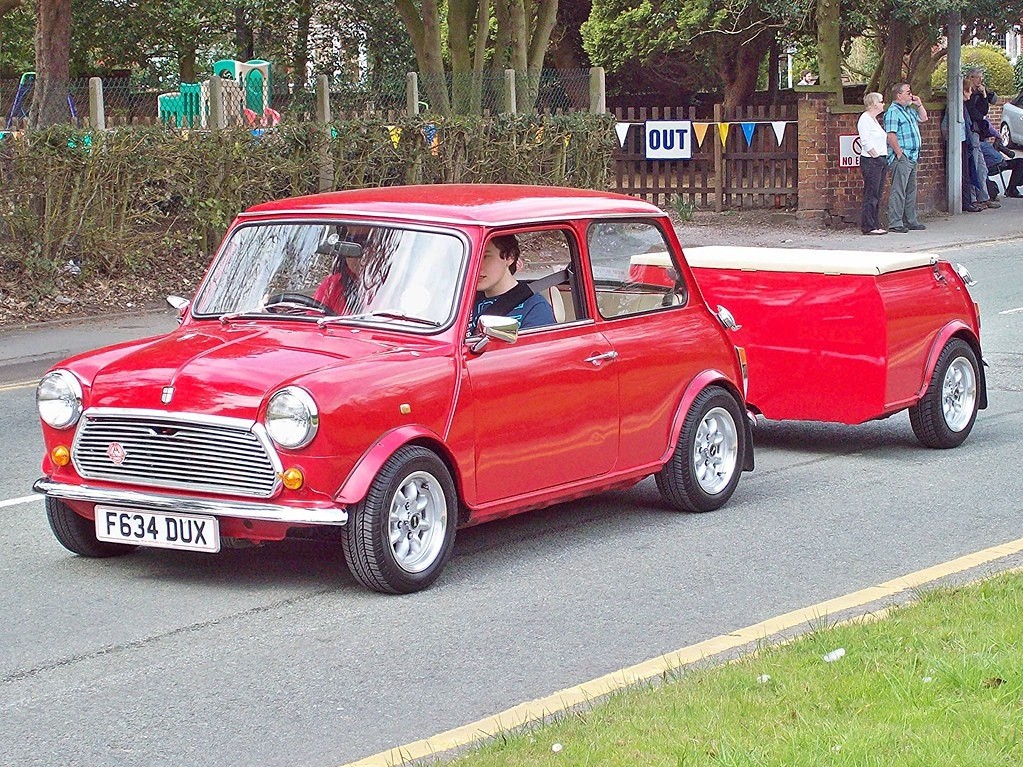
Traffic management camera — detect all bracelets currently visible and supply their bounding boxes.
[918,103,923,107]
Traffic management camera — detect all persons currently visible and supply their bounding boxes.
[306,224,394,317]
[438,233,556,337]
[883,82,929,232]
[856,92,889,235]
[797,71,820,85]
[942,68,1023,213]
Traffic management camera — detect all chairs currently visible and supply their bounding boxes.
[540,286,564,325]
[985,155,1009,197]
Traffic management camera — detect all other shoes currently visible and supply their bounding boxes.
[905,224,925,230]
[889,226,908,233]
[962,203,977,212]
[980,202,988,209]
[862,229,887,234]
[984,200,1001,208]
[1004,186,1023,198]
[971,202,983,211]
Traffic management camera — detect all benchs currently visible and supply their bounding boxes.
[561,290,682,323]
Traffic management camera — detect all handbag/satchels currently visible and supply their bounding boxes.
[970,121,980,132]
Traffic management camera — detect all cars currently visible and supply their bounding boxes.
[999,90,1023,150]
[33,182,758,597]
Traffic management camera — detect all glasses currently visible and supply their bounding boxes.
[343,235,376,248]
[877,101,884,104]
